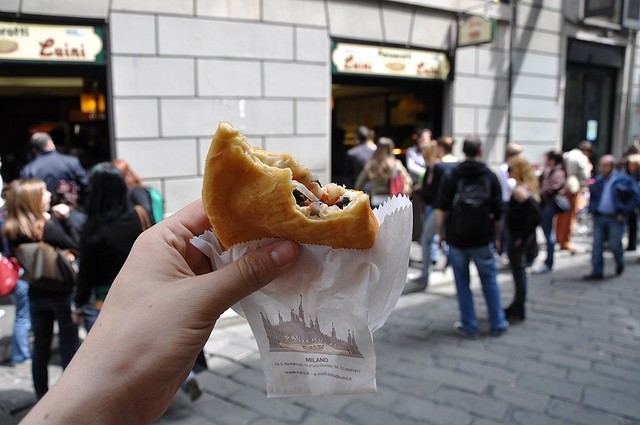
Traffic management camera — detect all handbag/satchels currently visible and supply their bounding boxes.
[552,191,571,214]
[390,175,406,196]
[17,241,76,288]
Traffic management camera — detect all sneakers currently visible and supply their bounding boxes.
[453,321,478,338]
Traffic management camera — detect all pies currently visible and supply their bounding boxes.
[202,120,377,251]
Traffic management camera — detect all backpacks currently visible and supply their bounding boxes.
[445,179,496,250]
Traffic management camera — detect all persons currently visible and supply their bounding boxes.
[625,154,639,251]
[353,137,413,208]
[495,143,522,264]
[419,142,439,238]
[19,132,87,193]
[114,160,153,227]
[73,162,152,331]
[18,199,301,425]
[507,155,542,320]
[619,142,639,172]
[409,137,458,282]
[438,135,509,335]
[344,126,377,188]
[9,181,80,399]
[406,128,434,242]
[0,175,31,365]
[562,140,593,249]
[584,155,639,280]
[539,152,567,273]
